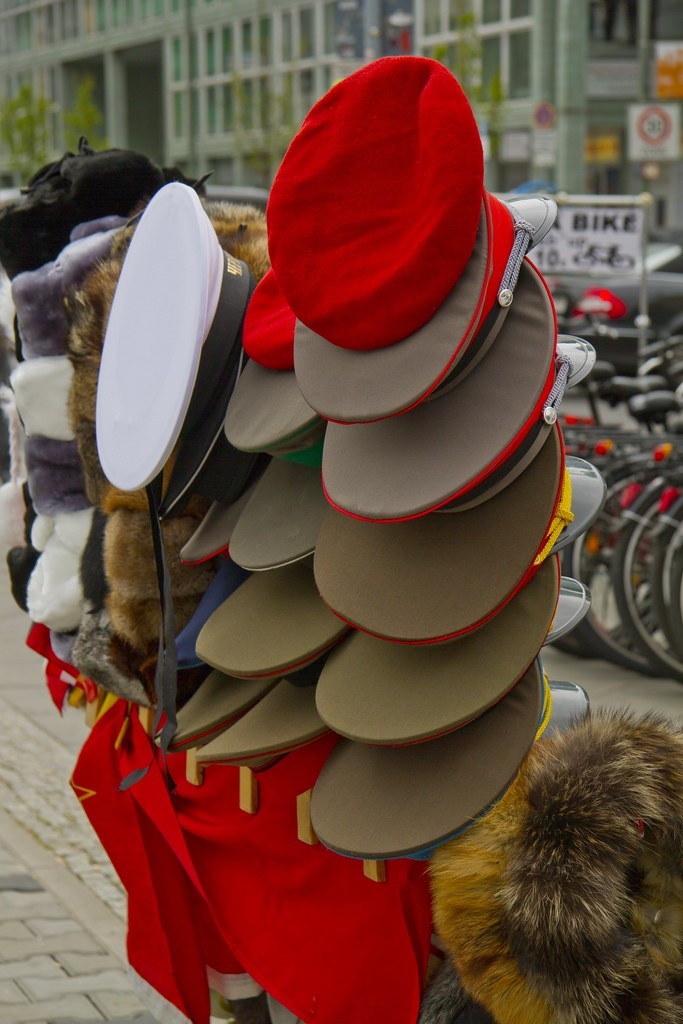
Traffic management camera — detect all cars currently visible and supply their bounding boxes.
[555,243,683,374]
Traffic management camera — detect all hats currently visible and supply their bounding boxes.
[95,61,601,862]
[0,144,205,706]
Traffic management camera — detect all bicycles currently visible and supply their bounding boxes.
[547,312,683,681]
